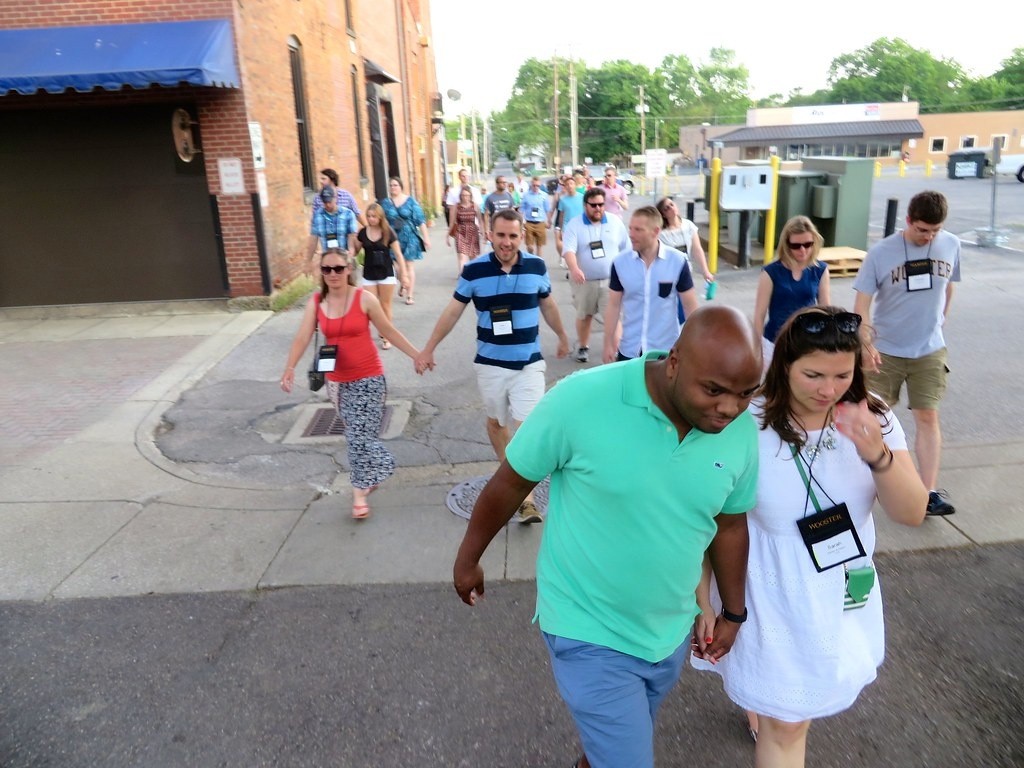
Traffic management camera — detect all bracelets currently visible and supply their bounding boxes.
[721,605,747,622]
[287,367,294,369]
[863,443,893,472]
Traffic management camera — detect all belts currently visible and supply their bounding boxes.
[526,221,539,224]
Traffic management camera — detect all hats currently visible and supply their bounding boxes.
[320,186,334,202]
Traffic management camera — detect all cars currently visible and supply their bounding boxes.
[587,167,636,195]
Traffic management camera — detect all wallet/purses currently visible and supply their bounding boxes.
[706,282,717,299]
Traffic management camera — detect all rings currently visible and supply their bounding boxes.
[281,382,283,385]
[862,425,866,430]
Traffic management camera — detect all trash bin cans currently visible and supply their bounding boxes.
[757,169,827,248]
[949,151,985,178]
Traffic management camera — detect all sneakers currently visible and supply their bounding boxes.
[926,488,956,516]
[518,502,543,525]
[578,347,589,362]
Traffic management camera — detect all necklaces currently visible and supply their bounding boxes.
[788,418,837,461]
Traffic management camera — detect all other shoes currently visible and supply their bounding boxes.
[748,729,758,742]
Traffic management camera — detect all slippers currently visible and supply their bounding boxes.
[351,505,369,519]
[405,296,415,304]
[365,484,378,494]
[381,339,391,350]
[399,286,406,297]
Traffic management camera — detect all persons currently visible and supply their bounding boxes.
[853,190,961,515]
[755,215,832,386]
[697,154,707,168]
[690,307,930,768]
[441,167,630,279]
[304,186,357,279]
[656,197,714,325]
[415,209,568,523]
[381,176,430,305]
[562,187,629,363]
[453,305,764,768]
[281,248,436,519]
[311,169,368,226]
[603,206,698,365]
[355,202,408,349]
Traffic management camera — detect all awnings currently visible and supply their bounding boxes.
[0,20,242,97]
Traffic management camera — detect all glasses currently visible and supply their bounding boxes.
[320,265,347,274]
[586,202,604,208]
[787,240,815,249]
[663,202,674,211]
[798,312,863,334]
[605,175,611,178]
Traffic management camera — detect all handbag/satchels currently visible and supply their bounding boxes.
[308,369,324,391]
[416,236,426,252]
